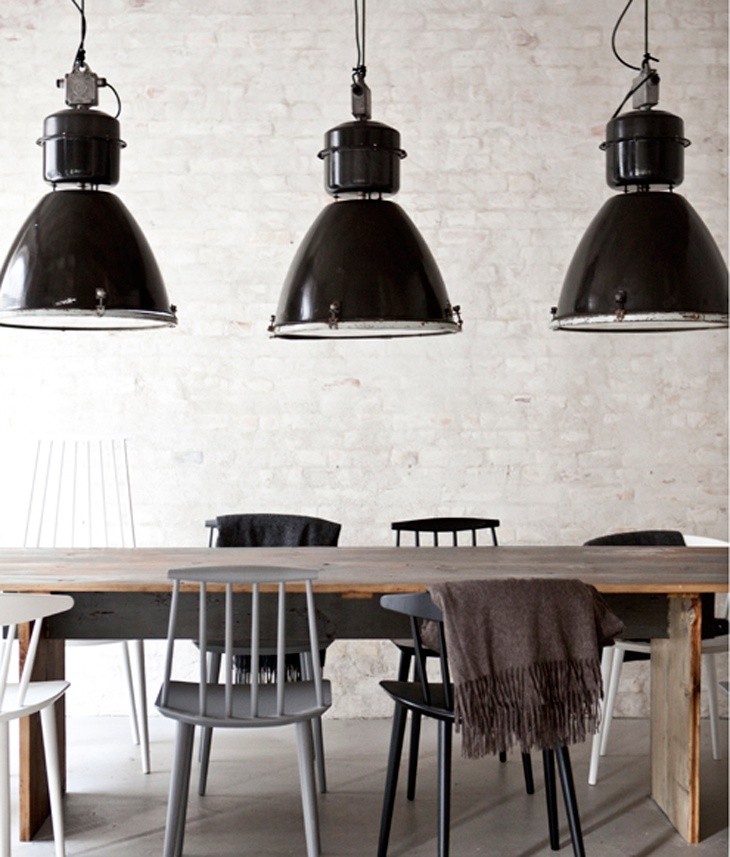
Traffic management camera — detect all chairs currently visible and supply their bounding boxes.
[20,440,153,773]
[377,578,585,857]
[199,514,342,762]
[585,530,730,785]
[0,593,76,857]
[155,566,332,857]
[391,516,534,801]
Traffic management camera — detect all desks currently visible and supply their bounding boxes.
[0,545,729,843]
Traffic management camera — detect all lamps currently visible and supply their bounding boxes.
[0,0,178,331]
[270,1,464,337]
[550,0,730,332]
